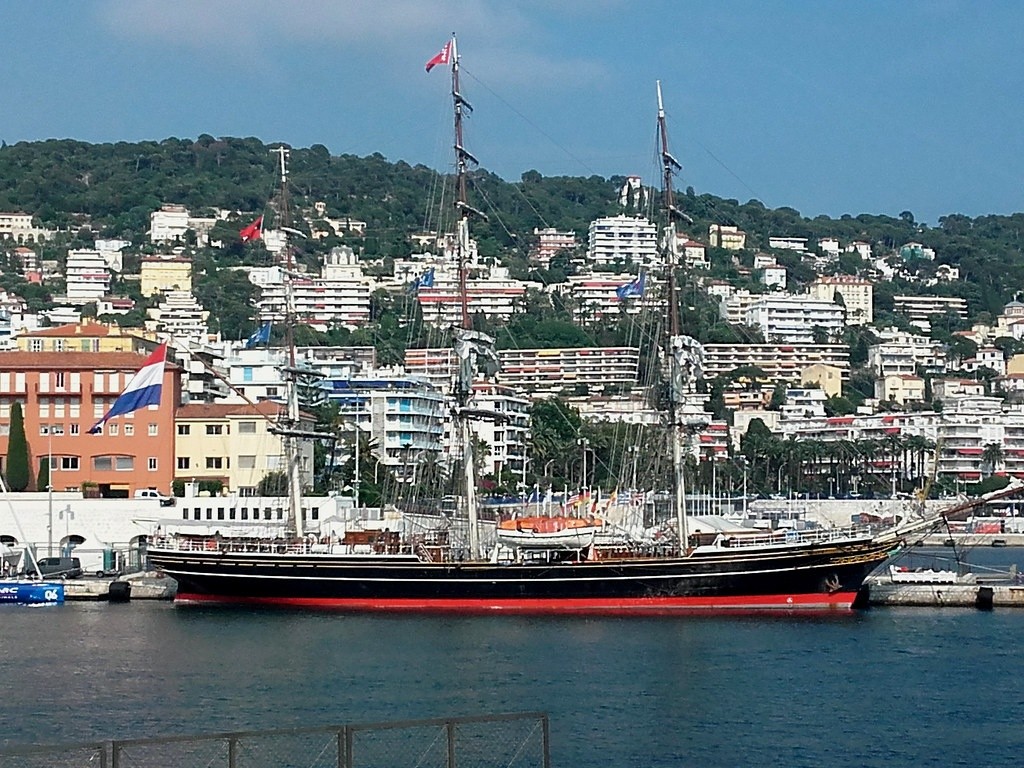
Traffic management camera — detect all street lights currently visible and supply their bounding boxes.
[41,425,56,558]
[743,458,751,519]
[709,455,720,516]
[544,458,556,478]
[576,437,591,501]
[778,461,787,494]
[523,458,536,485]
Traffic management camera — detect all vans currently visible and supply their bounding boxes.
[28,556,82,580]
[135,488,166,498]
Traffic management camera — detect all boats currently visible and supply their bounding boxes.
[888,564,958,585]
[495,513,616,558]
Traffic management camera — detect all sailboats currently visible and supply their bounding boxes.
[145,34,1024,619]
[0,476,65,607]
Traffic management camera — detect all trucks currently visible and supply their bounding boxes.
[71,548,126,578]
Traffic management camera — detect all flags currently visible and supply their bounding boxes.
[85,340,167,434]
[425,38,453,74]
[240,216,262,243]
[246,320,270,351]
[410,267,434,289]
[615,267,645,298]
